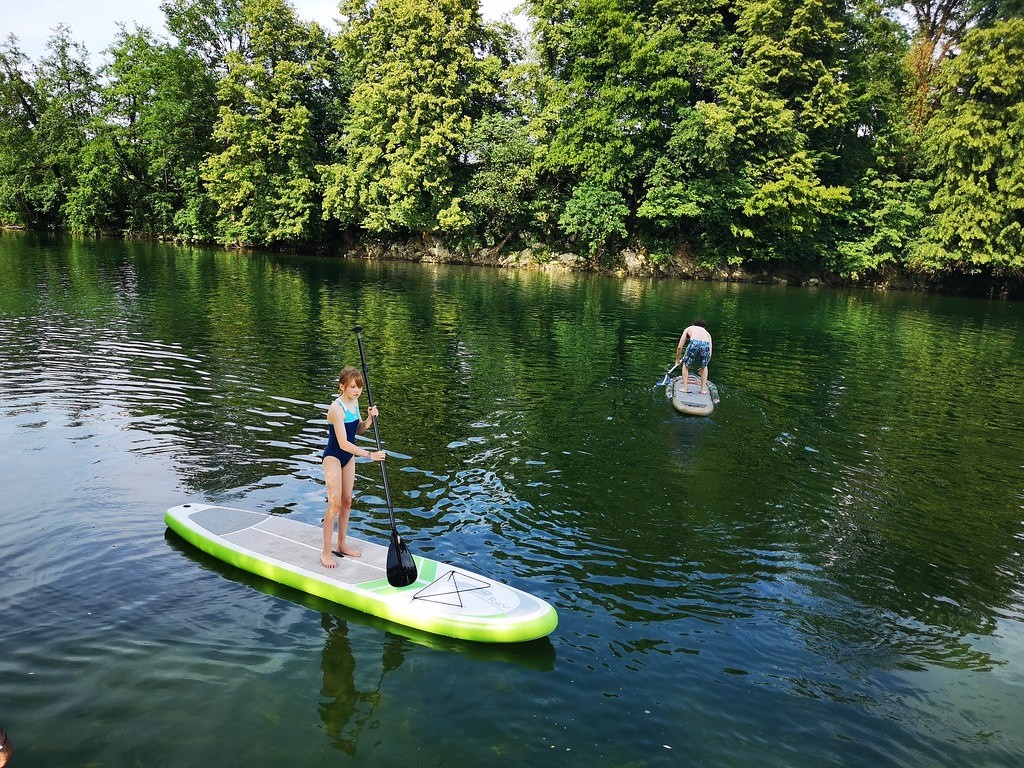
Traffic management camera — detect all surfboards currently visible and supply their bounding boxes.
[161,501,560,645]
[671,376,715,417]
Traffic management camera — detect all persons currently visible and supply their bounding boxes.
[677,320,712,394]
[321,367,386,568]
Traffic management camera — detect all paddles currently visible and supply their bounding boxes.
[350,324,419,588]
[656,356,685,387]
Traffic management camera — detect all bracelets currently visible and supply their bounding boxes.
[367,451,370,458]
